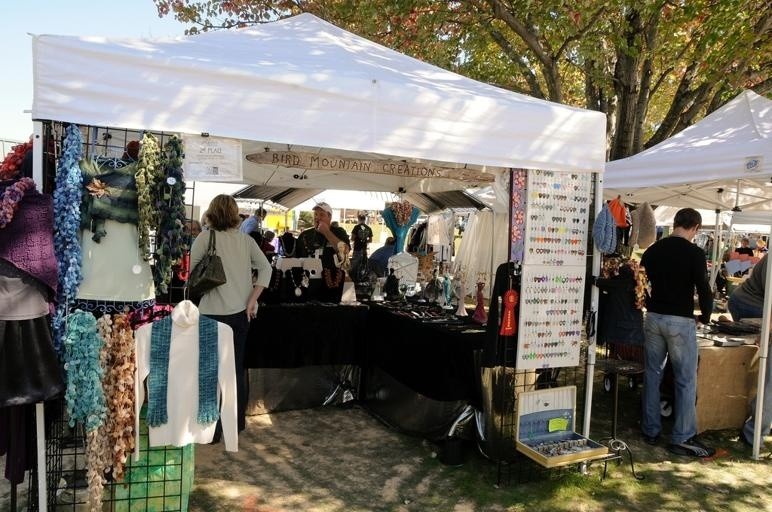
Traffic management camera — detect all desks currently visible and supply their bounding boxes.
[697,333,761,432]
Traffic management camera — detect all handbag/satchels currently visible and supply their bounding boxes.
[191,230,226,296]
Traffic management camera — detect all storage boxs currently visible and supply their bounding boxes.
[514,385,609,470]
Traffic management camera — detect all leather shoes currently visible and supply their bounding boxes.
[739,431,754,448]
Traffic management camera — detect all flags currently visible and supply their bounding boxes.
[723,251,762,276]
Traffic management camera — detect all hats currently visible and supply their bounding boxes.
[311,202,333,213]
[609,195,630,227]
[594,206,618,254]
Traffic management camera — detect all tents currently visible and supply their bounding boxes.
[568,89,771,462]
[25,14,614,498]
[624,205,772,291]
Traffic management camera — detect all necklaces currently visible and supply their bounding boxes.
[301,231,327,254]
[267,269,282,293]
[322,268,343,289]
[356,257,375,282]
[281,236,295,255]
[290,268,306,296]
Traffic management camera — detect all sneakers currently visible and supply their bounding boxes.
[645,434,661,445]
[667,437,716,457]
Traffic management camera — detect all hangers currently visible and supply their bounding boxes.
[173,287,193,318]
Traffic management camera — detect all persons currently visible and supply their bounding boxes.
[260,231,276,252]
[383,268,401,299]
[423,271,443,302]
[371,237,396,274]
[735,238,754,256]
[453,277,468,316]
[334,241,352,281]
[298,201,351,257]
[238,208,268,234]
[641,207,718,460]
[176,220,201,281]
[472,282,488,322]
[350,215,372,259]
[440,275,450,305]
[728,253,769,322]
[185,193,273,446]
